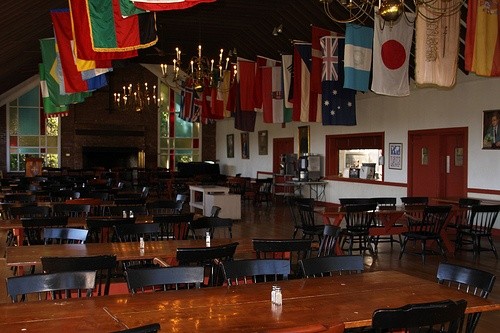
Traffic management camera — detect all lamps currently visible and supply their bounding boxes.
[277,25,284,32]
[158,6,238,91]
[319,0,471,29]
[272,28,278,35]
[113,79,160,110]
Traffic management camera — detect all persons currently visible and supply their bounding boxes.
[485,115,500,146]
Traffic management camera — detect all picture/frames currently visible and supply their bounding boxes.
[258,130,268,155]
[240,132,250,159]
[297,126,309,158]
[226,135,234,158]
[482,109,500,150]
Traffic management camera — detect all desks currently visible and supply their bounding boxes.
[285,181,328,200]
[314,198,466,262]
[0,237,319,276]
[0,215,203,246]
[432,198,500,222]
[190,184,229,216]
[0,269,500,333]
[0,199,153,216]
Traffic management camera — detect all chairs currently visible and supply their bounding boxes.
[0,169,500,333]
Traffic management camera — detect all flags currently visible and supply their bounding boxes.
[415,0,461,90]
[39,0,216,118]
[261,66,284,123]
[310,27,344,94]
[465,0,500,77]
[371,6,416,97]
[280,54,295,123]
[343,24,373,93]
[292,43,322,123]
[178,56,256,132]
[254,56,281,113]
[319,36,357,126]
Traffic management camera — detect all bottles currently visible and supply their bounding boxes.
[270,286,277,303]
[130,211,134,218]
[275,288,282,305]
[123,211,127,218]
[140,238,144,249]
[206,232,210,243]
